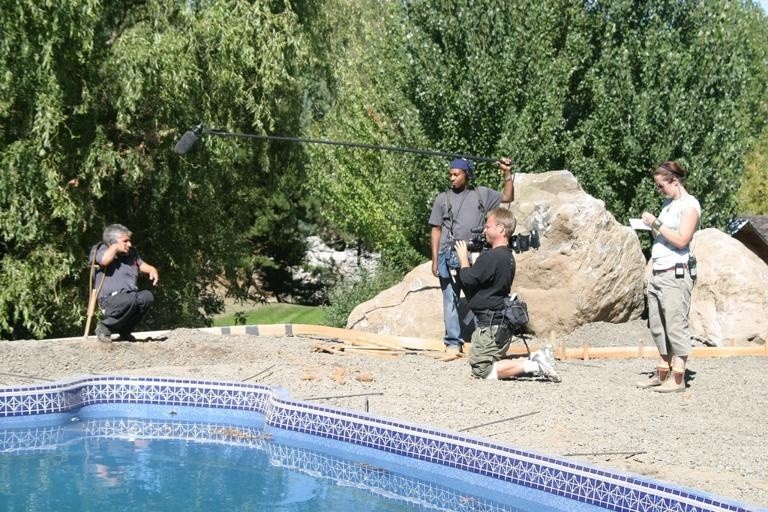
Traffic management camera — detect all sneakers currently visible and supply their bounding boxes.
[434,347,461,362]
[95,322,113,344]
[534,342,563,376]
[532,353,561,383]
[119,331,135,343]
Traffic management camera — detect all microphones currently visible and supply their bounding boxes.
[174,132,199,155]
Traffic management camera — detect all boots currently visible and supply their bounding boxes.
[637,367,670,389]
[654,369,687,393]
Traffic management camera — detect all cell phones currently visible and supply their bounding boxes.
[110,239,117,245]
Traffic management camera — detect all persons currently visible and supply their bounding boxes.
[88,223,160,344]
[636,158,702,393]
[429,156,516,358]
[454,207,563,381]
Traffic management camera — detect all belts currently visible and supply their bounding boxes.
[660,262,689,271]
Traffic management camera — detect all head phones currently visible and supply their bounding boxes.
[455,158,474,180]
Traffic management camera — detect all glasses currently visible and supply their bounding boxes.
[653,183,672,188]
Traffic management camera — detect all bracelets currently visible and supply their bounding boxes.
[503,174,512,182]
[650,218,663,230]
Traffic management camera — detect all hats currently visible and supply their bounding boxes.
[450,159,469,172]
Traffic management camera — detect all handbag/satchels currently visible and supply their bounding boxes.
[502,295,534,336]
